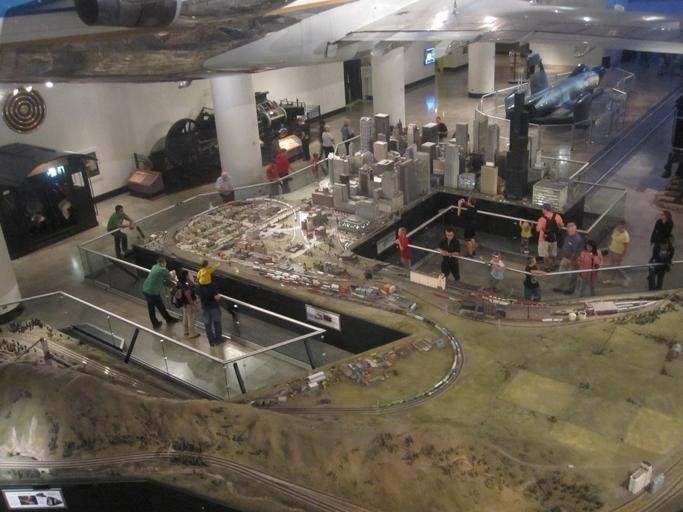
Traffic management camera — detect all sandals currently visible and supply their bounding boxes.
[550,266,555,271]
[540,266,551,273]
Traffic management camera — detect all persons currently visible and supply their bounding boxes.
[107,205,135,256]
[435,117,448,141]
[318,119,326,155]
[271,132,287,163]
[275,148,290,192]
[143,257,180,328]
[322,125,336,168]
[394,227,411,268]
[196,278,228,345]
[519,219,533,253]
[552,219,585,295]
[196,259,221,286]
[215,172,235,203]
[438,225,460,281]
[302,129,311,161]
[169,261,195,321]
[457,196,478,258]
[577,240,603,297]
[648,210,673,263]
[523,255,547,302]
[536,204,564,272]
[648,236,674,291]
[267,159,279,194]
[603,220,632,286]
[340,119,355,155]
[177,270,201,338]
[488,251,505,292]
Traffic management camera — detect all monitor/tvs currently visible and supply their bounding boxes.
[423,47,437,65]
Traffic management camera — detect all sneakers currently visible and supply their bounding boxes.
[552,287,564,293]
[153,321,162,329]
[166,317,178,323]
[210,341,216,346]
[603,279,616,286]
[189,333,201,339]
[563,290,574,296]
[622,276,632,287]
[184,332,189,336]
[216,338,228,345]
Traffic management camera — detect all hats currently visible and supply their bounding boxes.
[542,204,551,210]
